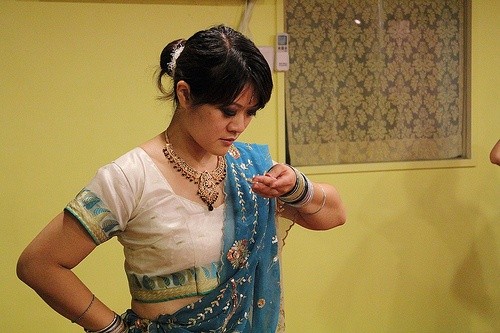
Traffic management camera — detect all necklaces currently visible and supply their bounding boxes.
[162,130,226,211]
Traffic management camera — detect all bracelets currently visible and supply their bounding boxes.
[299,184,327,216]
[266,164,314,208]
[84,312,129,333]
[72,294,95,323]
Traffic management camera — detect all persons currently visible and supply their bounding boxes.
[16,24,346,333]
